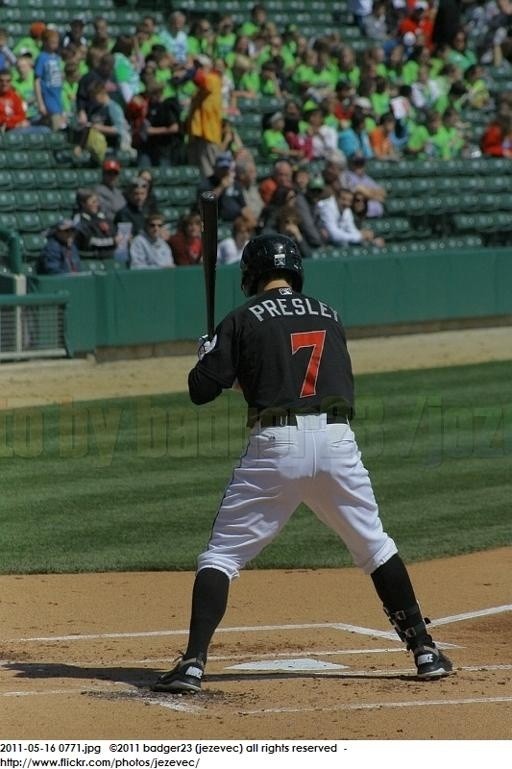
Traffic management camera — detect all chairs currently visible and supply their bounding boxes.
[0,2,511,277]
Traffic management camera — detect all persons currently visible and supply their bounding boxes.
[150,232,454,694]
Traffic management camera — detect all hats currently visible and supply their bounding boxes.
[56,219,78,229]
[308,177,325,189]
[103,160,119,173]
[215,154,231,169]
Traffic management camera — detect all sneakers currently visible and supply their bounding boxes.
[414,645,456,679]
[150,651,206,692]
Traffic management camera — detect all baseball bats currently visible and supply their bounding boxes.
[201,191,218,341]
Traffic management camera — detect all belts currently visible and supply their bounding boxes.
[250,411,347,429]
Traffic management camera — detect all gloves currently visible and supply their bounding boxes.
[196,335,217,359]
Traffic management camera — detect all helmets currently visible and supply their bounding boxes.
[241,233,304,297]
